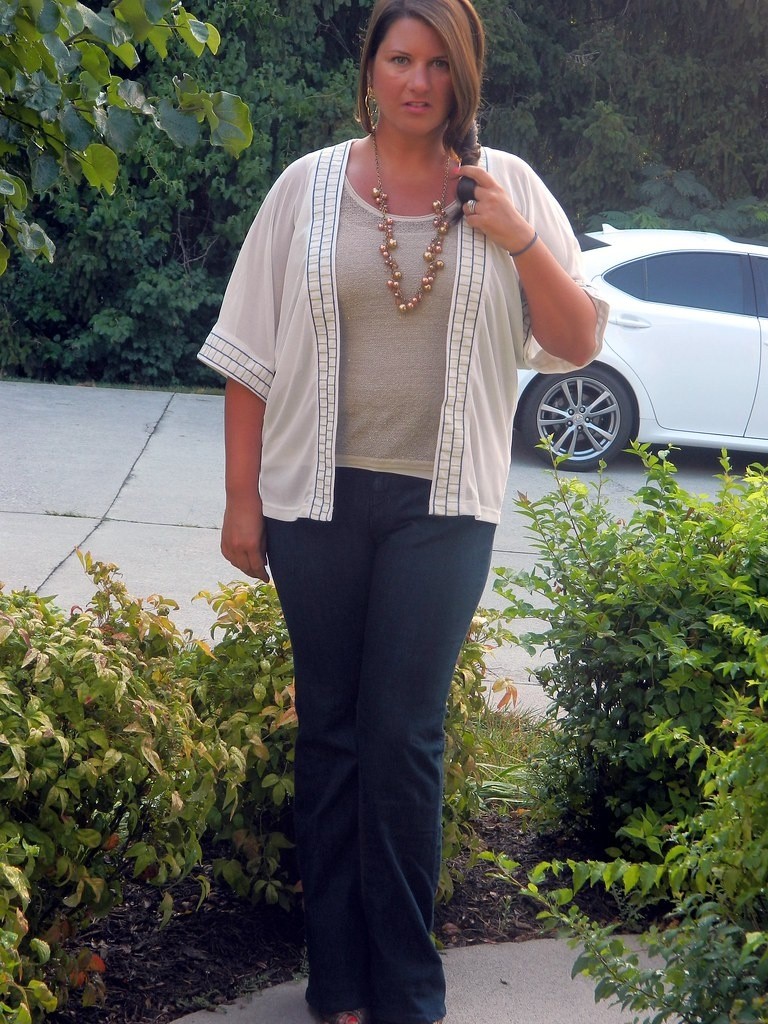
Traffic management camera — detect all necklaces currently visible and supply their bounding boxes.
[372,123,450,313]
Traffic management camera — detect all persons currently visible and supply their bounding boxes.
[197,0,612,1024]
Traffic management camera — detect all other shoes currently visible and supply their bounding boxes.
[323,1008,365,1024]
[433,1020,442,1024]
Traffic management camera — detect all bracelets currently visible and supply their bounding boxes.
[509,231,539,257]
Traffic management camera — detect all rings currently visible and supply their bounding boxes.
[467,200,477,214]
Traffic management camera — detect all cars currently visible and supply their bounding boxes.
[512,221,768,473]
